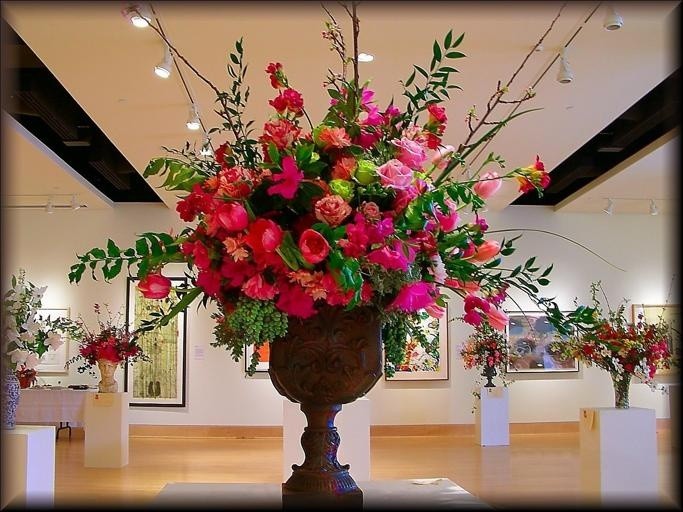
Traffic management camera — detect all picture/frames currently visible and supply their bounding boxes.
[385,302,449,381]
[124,277,187,407]
[505,311,579,373]
[245,339,270,372]
[31,306,70,373]
[633,304,682,377]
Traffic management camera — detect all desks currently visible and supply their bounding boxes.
[15,387,100,441]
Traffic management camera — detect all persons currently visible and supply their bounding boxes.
[98,359,118,393]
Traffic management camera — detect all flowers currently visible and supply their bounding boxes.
[0,266,73,374]
[549,279,683,395]
[64,1,647,383]
[449,314,518,414]
[59,302,155,379]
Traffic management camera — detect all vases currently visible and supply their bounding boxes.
[269,305,382,510]
[610,371,632,409]
[0,367,21,430]
[480,364,499,387]
